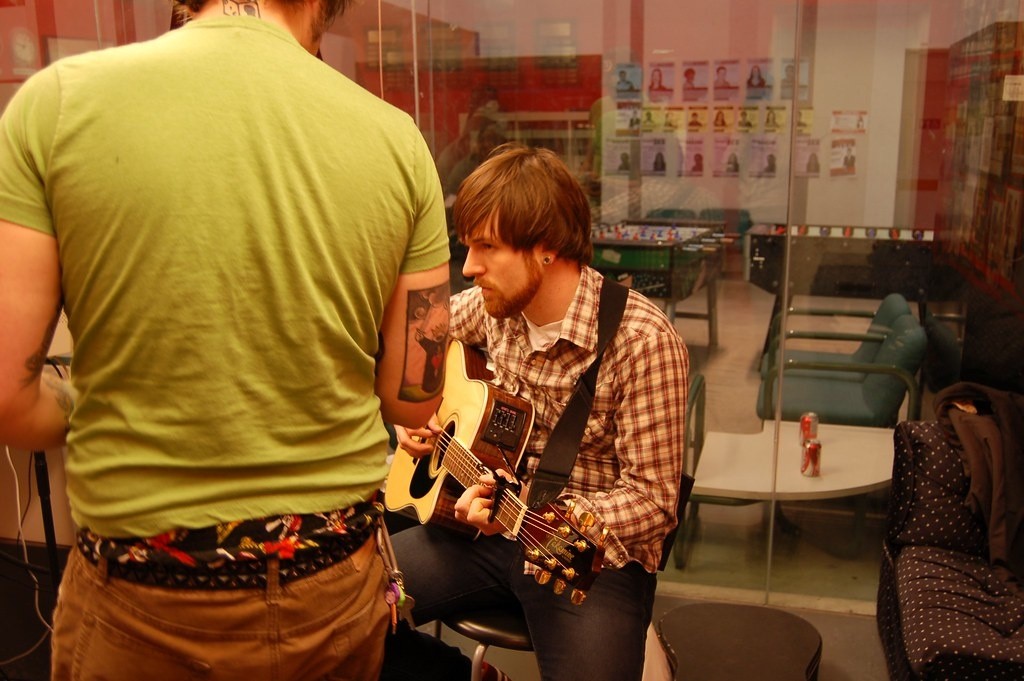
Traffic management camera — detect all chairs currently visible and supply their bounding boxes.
[924,316,1024,478]
[757,314,928,428]
[631,209,697,303]
[700,207,754,281]
[759,292,910,382]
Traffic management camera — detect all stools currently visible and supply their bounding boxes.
[435,612,534,681]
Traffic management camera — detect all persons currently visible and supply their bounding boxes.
[664,113,677,128]
[0,0,453,681]
[616,70,635,93]
[649,68,668,91]
[806,153,820,173]
[630,111,640,127]
[618,153,630,170]
[725,153,740,173]
[683,68,695,90]
[713,66,730,90]
[384,143,691,681]
[780,65,796,88]
[713,110,728,126]
[747,65,766,88]
[691,153,703,172]
[688,112,702,127]
[653,153,666,172]
[843,148,855,166]
[764,110,780,128]
[643,112,656,125]
[763,153,777,173]
[796,111,807,126]
[737,111,753,127]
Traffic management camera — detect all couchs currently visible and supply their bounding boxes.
[876,421,1024,681]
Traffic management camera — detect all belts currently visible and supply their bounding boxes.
[76,521,373,591]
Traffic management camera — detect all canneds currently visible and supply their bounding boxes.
[800,438,821,477]
[798,411,818,446]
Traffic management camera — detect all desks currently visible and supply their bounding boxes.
[589,222,720,357]
[687,419,896,561]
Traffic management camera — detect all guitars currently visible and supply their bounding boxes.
[385,339,609,610]
